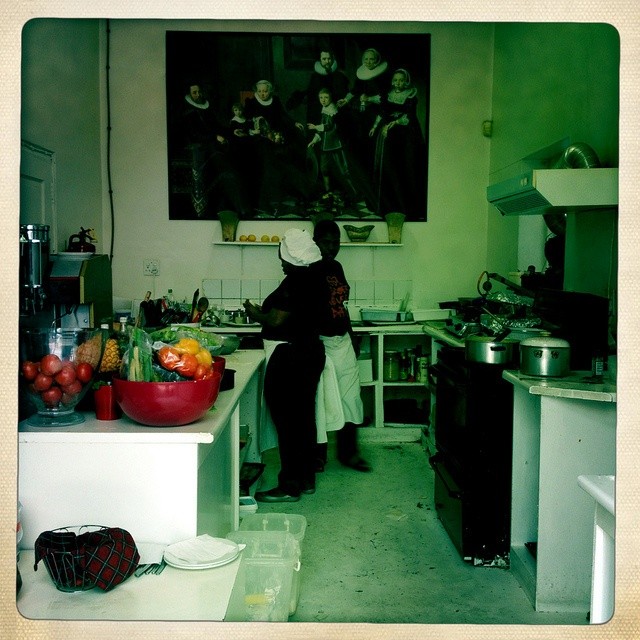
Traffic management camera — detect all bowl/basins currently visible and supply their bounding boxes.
[114,371,220,427]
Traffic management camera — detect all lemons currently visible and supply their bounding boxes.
[174,338,215,368]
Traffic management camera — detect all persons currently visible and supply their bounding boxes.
[244,79,306,153]
[307,88,351,201]
[312,219,374,472]
[243,228,326,503]
[336,48,390,115]
[228,102,262,138]
[178,83,227,151]
[367,69,425,216]
[307,48,349,103]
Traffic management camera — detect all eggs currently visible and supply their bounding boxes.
[261,235,270,242]
[239,235,248,241]
[271,236,280,242]
[248,235,256,241]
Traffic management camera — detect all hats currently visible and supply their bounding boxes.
[279,228,323,267]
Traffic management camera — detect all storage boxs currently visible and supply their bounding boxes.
[241,515,307,614]
[228,531,297,620]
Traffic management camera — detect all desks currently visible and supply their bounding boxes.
[17,545,242,621]
[578,474,615,624]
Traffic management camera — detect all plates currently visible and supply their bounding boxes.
[163,536,241,569]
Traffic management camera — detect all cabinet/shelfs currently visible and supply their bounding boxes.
[16,419,234,545]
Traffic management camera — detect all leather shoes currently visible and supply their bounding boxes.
[336,457,371,472]
[301,483,315,494]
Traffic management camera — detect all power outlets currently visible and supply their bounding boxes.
[144,259,159,276]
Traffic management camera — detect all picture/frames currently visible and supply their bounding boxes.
[165,29,431,224]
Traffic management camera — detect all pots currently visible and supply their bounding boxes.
[467,336,517,365]
[521,338,570,377]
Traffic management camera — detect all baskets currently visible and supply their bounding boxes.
[35,526,122,592]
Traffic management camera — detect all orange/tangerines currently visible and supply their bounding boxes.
[159,345,207,377]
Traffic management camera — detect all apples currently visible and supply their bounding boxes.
[22,356,88,409]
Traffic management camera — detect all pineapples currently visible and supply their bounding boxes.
[74,331,102,369]
[99,338,119,373]
